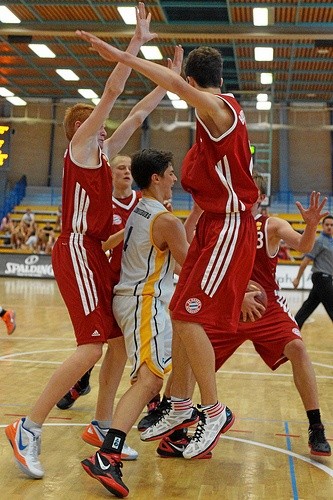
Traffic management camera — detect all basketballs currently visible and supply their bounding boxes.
[240,280,267,322]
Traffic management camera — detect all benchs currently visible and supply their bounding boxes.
[0,204,333,268]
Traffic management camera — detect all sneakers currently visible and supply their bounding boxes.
[82,421,139,460]
[182,404,235,460]
[147,394,160,413]
[56,381,91,410]
[308,424,332,457]
[5,417,45,479]
[137,399,171,431]
[1,309,16,335]
[80,450,130,499]
[140,400,200,442]
[157,436,212,459]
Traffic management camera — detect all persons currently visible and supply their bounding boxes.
[80,149,204,498]
[76,29,259,460]
[5,2,184,479]
[138,172,333,461]
[55,154,173,410]
[294,215,333,332]
[0,205,64,254]
